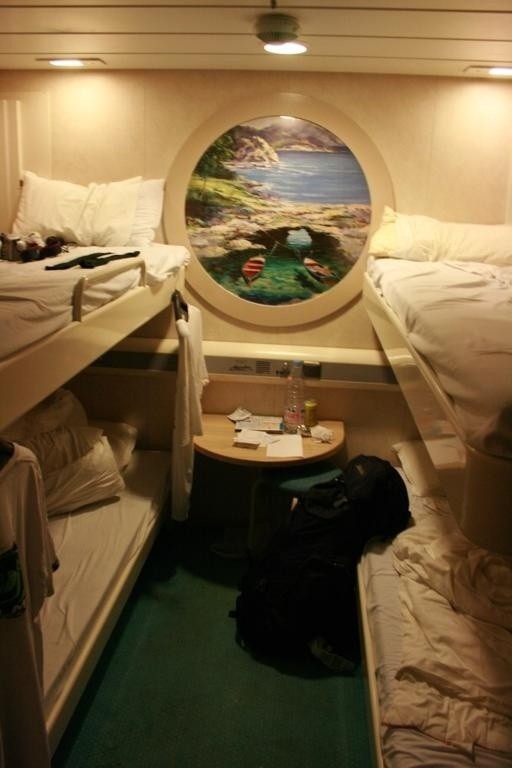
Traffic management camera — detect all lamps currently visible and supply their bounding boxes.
[253,12,300,46]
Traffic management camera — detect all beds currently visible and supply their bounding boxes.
[0,242,191,768]
[357,257,512,768]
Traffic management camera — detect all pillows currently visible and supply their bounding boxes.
[366,203,512,267]
[88,420,140,473]
[389,439,447,499]
[8,169,166,248]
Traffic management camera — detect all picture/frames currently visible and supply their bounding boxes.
[161,93,398,329]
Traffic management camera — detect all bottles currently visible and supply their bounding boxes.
[306,401,317,425]
[283,360,304,431]
